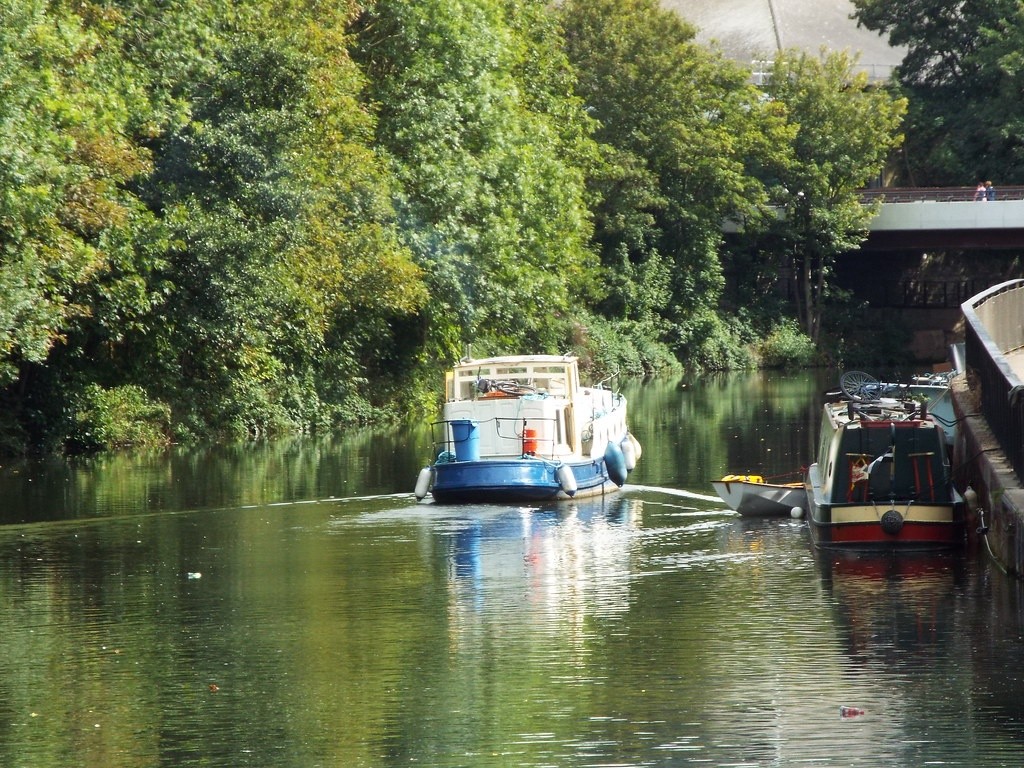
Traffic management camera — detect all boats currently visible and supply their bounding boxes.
[430,344,629,500]
[711,470,806,516]
[804,396,969,553]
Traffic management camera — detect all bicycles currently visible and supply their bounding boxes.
[839,371,910,404]
